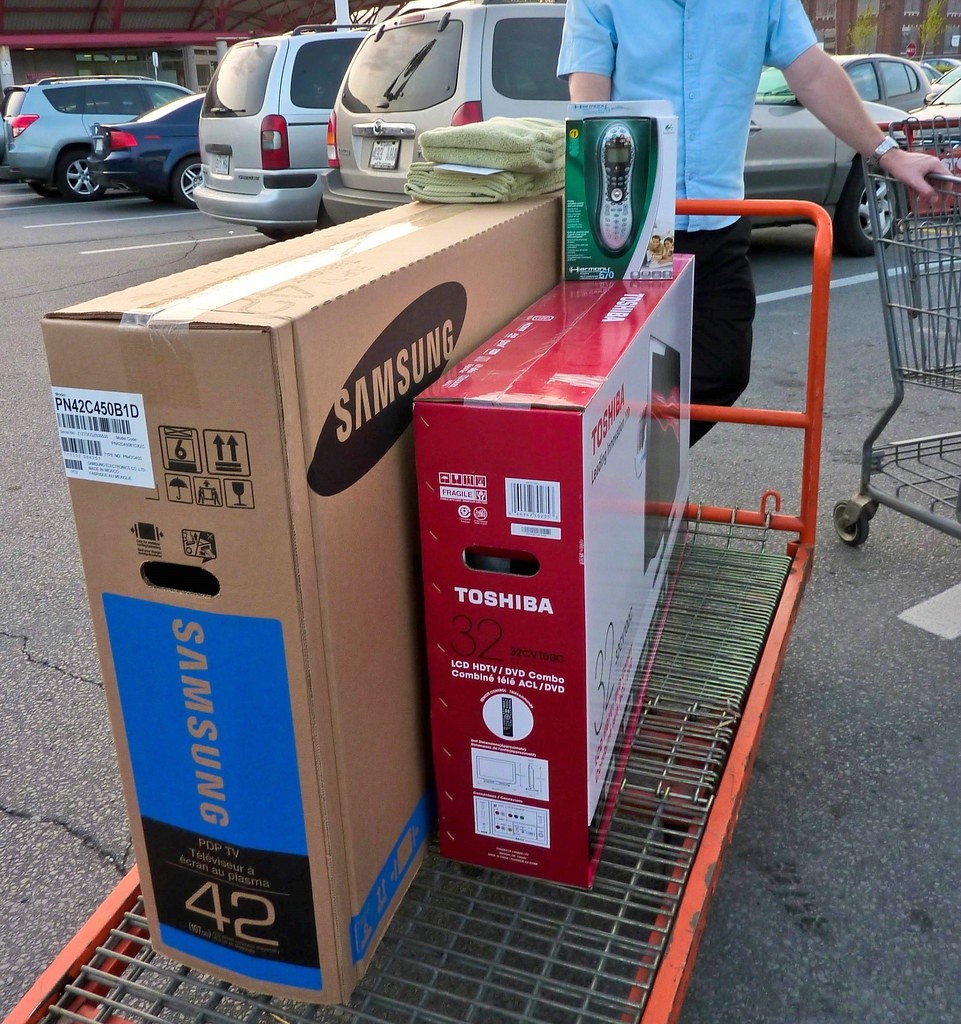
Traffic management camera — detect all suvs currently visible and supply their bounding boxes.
[0,75,196,203]
[193,0,916,257]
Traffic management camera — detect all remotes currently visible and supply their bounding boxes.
[599,123,635,253]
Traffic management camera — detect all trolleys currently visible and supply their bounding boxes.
[0,199,835,1024]
[831,116,961,545]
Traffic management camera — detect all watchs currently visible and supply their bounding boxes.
[870,136,899,167]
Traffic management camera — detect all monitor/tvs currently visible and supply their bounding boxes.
[642,334,681,589]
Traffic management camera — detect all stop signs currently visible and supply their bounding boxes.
[906,44,917,59]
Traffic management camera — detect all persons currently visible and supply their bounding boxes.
[647,234,674,262]
[556,0,953,447]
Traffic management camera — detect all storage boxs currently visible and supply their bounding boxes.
[563,100,679,281]
[38,188,562,1007]
[415,254,699,892]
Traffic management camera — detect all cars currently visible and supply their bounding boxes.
[86,92,206,209]
[755,51,961,209]
